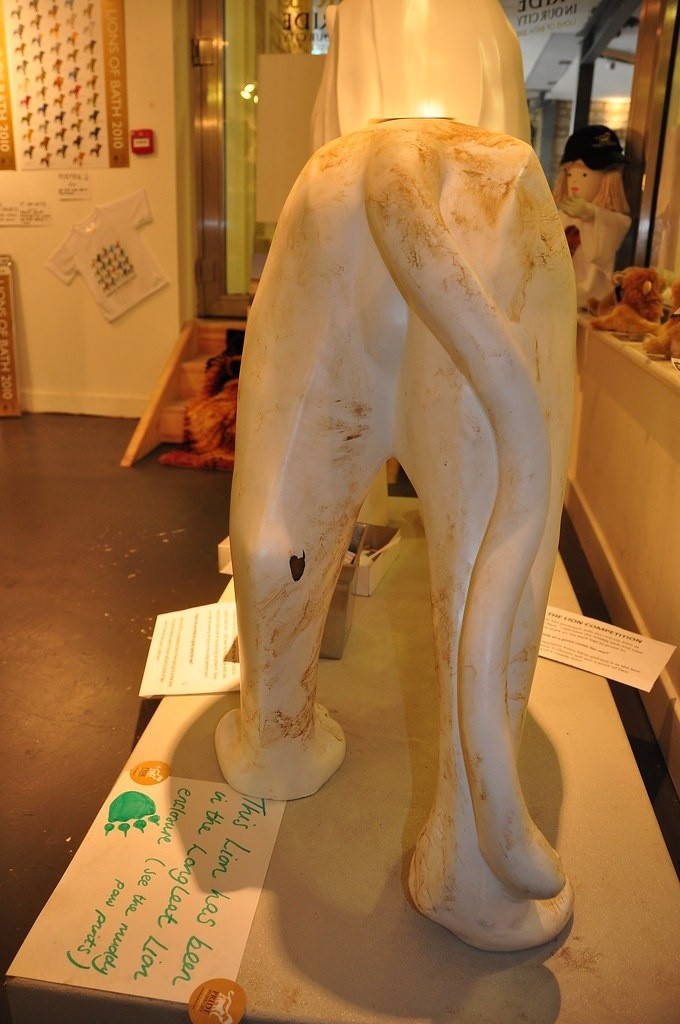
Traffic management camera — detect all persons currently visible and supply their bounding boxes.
[555,122,632,308]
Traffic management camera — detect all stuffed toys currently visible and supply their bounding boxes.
[644,281,680,359]
[593,265,661,331]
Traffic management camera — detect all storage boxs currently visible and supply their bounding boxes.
[218,522,405,659]
